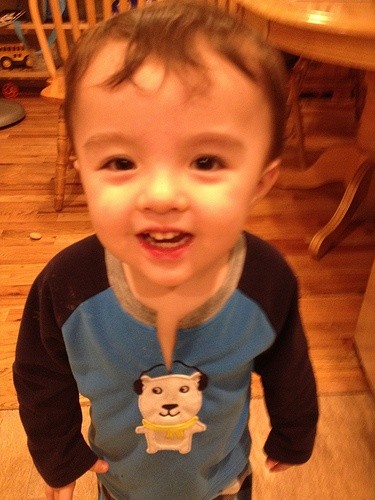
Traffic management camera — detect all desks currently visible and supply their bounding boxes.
[235,0,375,260]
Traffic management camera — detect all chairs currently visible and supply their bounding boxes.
[27,0,240,214]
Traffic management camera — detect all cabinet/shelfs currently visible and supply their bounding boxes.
[0,22,89,78]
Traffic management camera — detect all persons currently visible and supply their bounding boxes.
[12,0,320,500]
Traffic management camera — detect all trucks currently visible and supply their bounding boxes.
[0,42,36,69]
